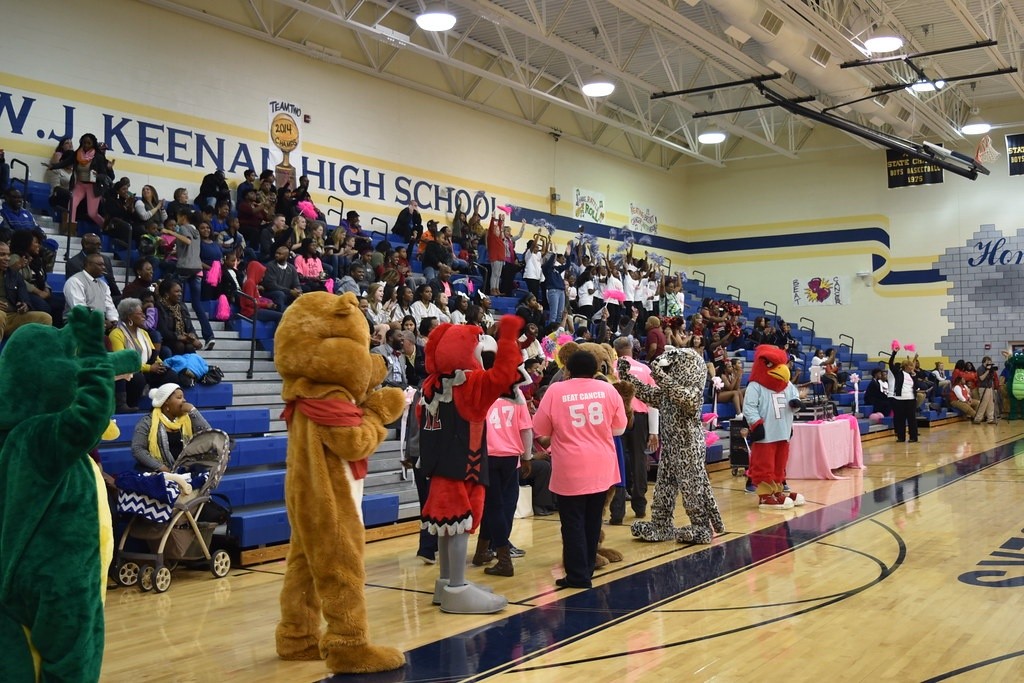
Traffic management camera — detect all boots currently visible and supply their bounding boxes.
[472,538,494,565]
[484,546,514,576]
[65,221,78,236]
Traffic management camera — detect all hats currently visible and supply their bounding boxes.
[98,142,108,149]
[148,383,180,408]
[347,211,360,219]
[427,220,439,227]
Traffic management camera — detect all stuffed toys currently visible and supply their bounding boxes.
[616,345,724,543]
[743,346,805,510]
[559,343,634,567]
[275,291,406,672]
[1006,349,1024,420]
[420,315,521,614]
[0,307,142,683]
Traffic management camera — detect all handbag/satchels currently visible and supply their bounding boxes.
[196,493,233,526]
[201,365,224,385]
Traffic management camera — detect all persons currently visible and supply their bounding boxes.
[474,384,534,575]
[48,132,694,429]
[534,352,628,588]
[130,382,213,476]
[750,316,801,385]
[610,337,659,527]
[399,364,439,564]
[0,148,214,591]
[950,357,1009,424]
[740,382,791,492]
[811,348,839,394]
[691,298,749,419]
[864,346,952,442]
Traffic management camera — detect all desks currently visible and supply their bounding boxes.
[783,412,867,480]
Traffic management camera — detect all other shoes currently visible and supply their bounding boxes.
[989,421,998,424]
[909,438,916,442]
[783,485,791,492]
[491,289,505,297]
[117,405,139,414]
[897,438,904,442]
[556,577,572,587]
[609,518,622,525]
[489,545,526,558]
[416,550,436,564]
[203,338,216,351]
[746,486,756,493]
[637,514,646,518]
[735,413,744,420]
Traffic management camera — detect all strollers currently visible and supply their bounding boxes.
[108,422,236,594]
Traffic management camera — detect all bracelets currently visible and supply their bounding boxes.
[190,407,195,412]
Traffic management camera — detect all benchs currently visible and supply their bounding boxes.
[0,176,961,547]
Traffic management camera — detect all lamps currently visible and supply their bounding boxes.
[864,0,902,52]
[698,120,726,144]
[415,0,457,32]
[581,65,615,97]
[960,82,991,135]
[912,25,943,92]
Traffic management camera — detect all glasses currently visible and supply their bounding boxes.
[93,262,105,267]
[133,311,144,316]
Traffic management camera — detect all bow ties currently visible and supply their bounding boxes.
[391,350,400,357]
